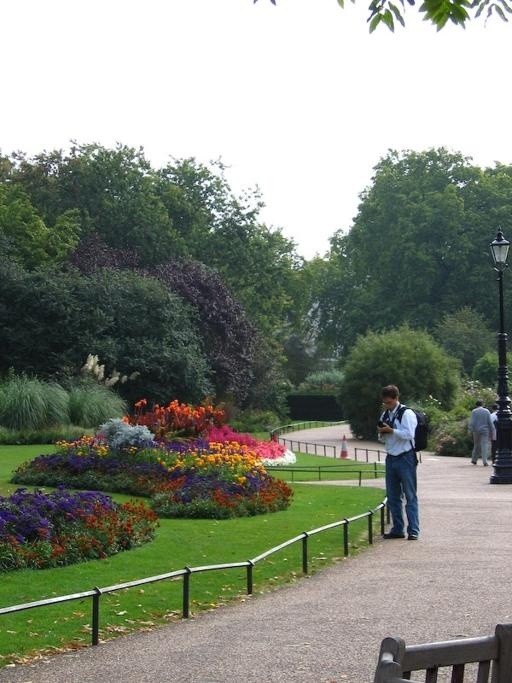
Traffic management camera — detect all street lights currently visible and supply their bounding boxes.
[490,226,512,484]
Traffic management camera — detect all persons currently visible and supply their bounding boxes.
[376,385,420,540]
[491,404,498,466]
[468,400,495,466]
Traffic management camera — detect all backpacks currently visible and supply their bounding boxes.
[397,407,428,451]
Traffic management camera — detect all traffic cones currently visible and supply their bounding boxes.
[338,435,352,460]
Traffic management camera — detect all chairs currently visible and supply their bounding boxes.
[375,621,512,683]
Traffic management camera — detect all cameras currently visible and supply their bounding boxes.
[378,421,392,428]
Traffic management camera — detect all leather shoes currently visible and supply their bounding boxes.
[383,534,404,539]
[485,463,488,466]
[472,461,476,464]
[408,534,417,540]
